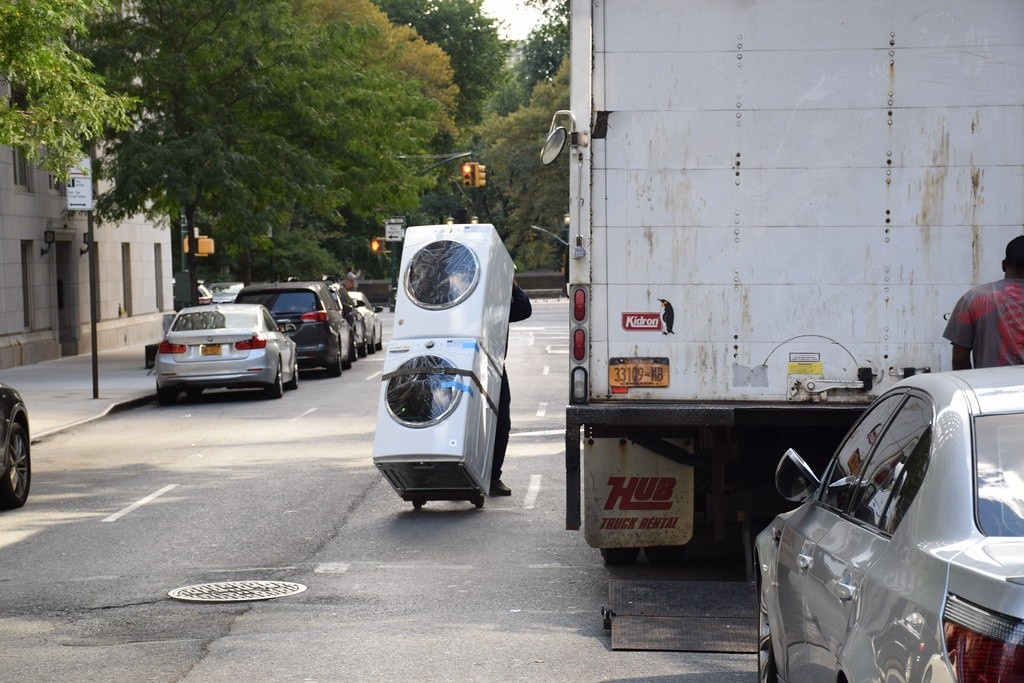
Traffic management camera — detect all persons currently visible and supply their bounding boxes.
[490,279,532,495]
[943,236,1024,371]
[346,267,361,291]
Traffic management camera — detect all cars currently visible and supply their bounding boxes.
[754,363,1024,683]
[155,303,299,399]
[238,281,354,377]
[0,380,31,510]
[336,290,383,353]
[285,282,368,361]
[203,280,243,303]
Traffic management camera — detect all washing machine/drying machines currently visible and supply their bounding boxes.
[391,223,515,379]
[371,338,502,503]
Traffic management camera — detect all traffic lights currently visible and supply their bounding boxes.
[478,165,488,187]
[461,162,477,189]
[370,240,379,252]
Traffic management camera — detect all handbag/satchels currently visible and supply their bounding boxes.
[345,280,354,289]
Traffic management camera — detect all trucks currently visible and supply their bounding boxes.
[540,0,1024,564]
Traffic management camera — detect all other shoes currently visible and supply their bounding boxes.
[490,479,511,495]
